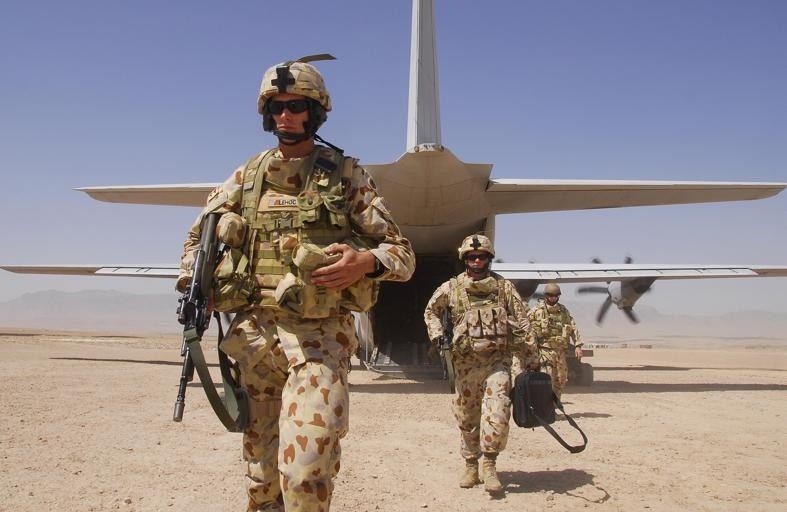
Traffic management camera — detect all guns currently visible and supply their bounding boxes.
[438,306,452,380]
[173,214,217,422]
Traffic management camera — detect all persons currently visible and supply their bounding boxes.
[176,62,416,512]
[424,234,537,492]
[511,301,532,388]
[527,284,584,400]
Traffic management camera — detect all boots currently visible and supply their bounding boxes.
[480,456,502,491]
[458,458,479,488]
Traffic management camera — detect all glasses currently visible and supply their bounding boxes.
[465,253,491,261]
[265,99,315,114]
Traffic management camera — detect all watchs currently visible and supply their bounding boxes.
[365,258,385,278]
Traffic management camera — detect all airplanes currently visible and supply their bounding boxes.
[0,0,787,379]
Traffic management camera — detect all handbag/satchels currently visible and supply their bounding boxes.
[510,365,556,429]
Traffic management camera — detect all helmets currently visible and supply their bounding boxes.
[543,284,561,295]
[256,59,332,116]
[458,234,495,261]
